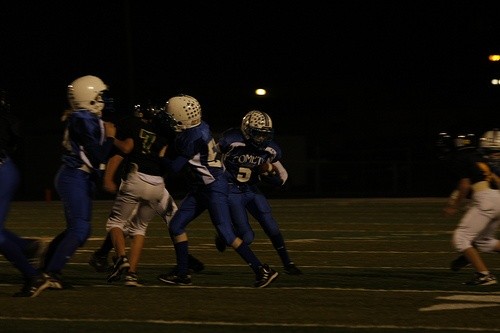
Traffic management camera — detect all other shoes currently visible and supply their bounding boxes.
[471,272,498,287]
[189,253,205,273]
[158,267,193,286]
[283,261,304,276]
[450,255,472,272]
[48,275,73,290]
[255,263,279,288]
[121,271,144,287]
[215,234,226,252]
[88,251,111,273]
[18,272,52,298]
[106,252,130,283]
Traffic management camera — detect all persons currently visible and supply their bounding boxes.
[0,74,304,299]
[443,131,500,288]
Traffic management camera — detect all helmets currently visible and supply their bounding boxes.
[134,103,159,120]
[66,75,108,114]
[478,130,500,152]
[163,95,202,130]
[241,110,273,148]
[453,133,477,150]
